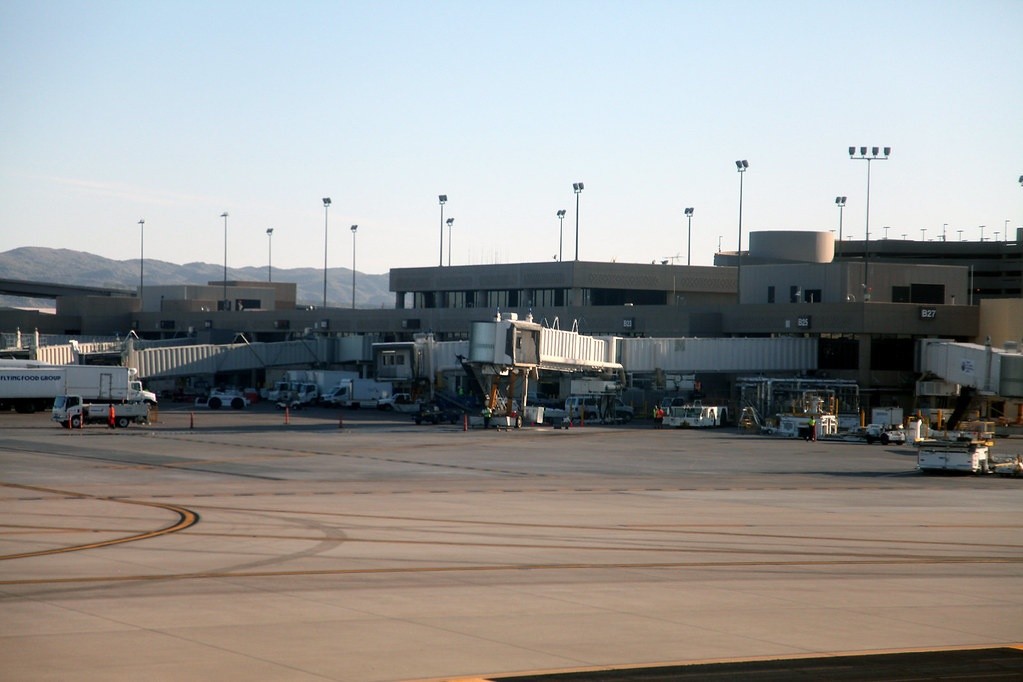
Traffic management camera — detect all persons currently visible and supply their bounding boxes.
[807,416,816,442]
[652,405,664,428]
[684,403,689,409]
[481,406,492,429]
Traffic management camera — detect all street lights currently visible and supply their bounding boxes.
[220,212,228,298]
[266,228,273,282]
[835,195,848,241]
[350,225,357,309]
[573,182,584,262]
[322,197,332,310]
[557,209,566,262]
[735,160,748,305]
[445,218,455,267]
[438,195,448,267]
[138,219,144,299]
[685,207,694,267]
[847,147,892,300]
[847,220,1011,242]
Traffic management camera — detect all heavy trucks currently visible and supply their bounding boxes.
[0,364,159,414]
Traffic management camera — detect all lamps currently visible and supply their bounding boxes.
[321,319,330,330]
[205,320,212,328]
[846,294,857,302]
[794,290,801,296]
[625,303,635,312]
[130,320,140,330]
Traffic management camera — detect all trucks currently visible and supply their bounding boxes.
[267,370,393,412]
[48,394,148,428]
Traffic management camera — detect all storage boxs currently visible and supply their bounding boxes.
[552,420,561,429]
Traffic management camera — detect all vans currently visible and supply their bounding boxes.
[565,396,634,423]
